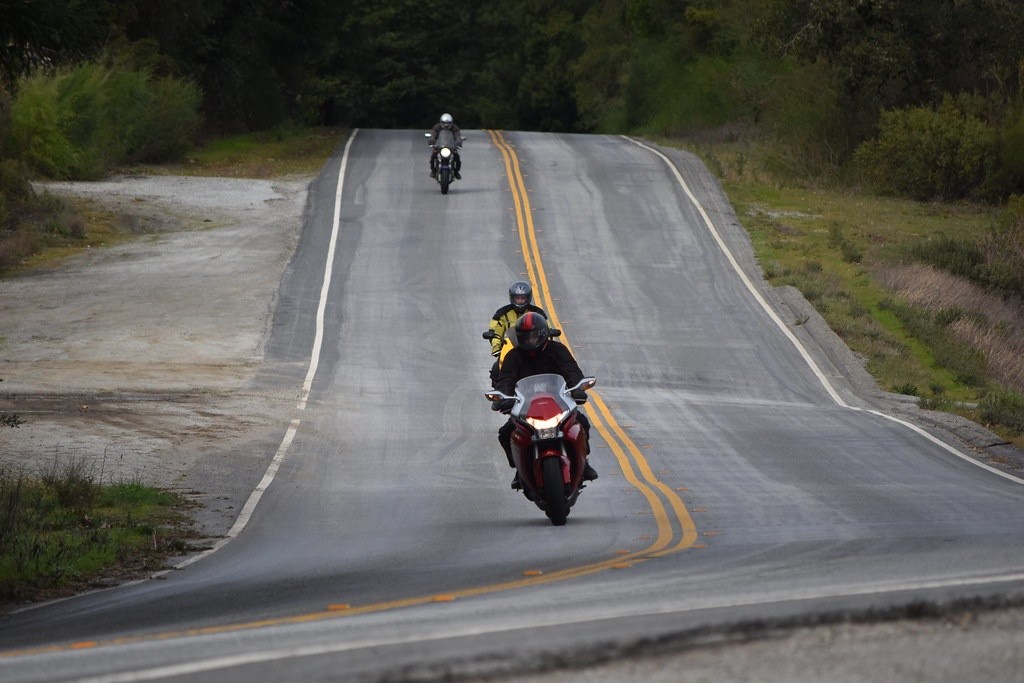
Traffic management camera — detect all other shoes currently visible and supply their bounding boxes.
[492,401,500,411]
[583,459,598,480]
[454,170,461,180]
[511,471,522,489]
[430,169,436,177]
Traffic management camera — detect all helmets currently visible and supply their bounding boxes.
[515,312,549,351]
[509,281,532,312]
[440,113,453,130]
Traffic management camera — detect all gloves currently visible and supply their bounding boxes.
[456,142,462,148]
[499,399,515,414]
[492,346,501,357]
[573,390,588,405]
[429,141,435,145]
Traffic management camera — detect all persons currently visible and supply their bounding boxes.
[489,281,553,356]
[428,113,462,180]
[492,312,598,489]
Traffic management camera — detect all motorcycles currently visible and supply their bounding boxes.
[482,329,561,370]
[425,133,467,194]
[485,374,596,525]
